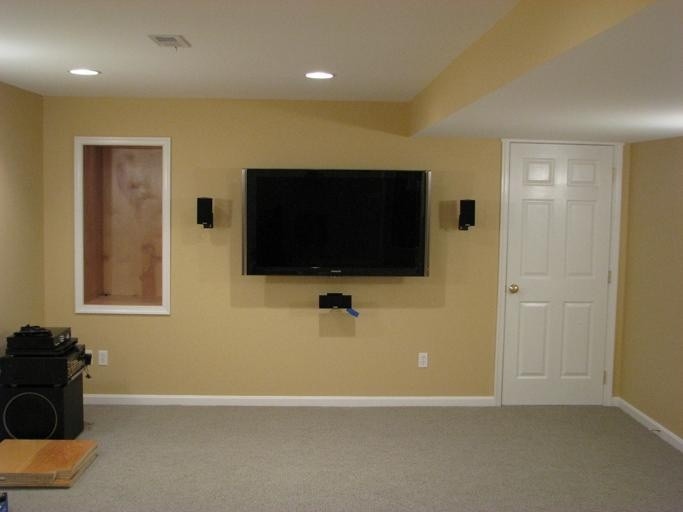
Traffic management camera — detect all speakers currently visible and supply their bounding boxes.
[196,197,214,229]
[319,293,352,309]
[458,199,476,230]
[0,370,85,443]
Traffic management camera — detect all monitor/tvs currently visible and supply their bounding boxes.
[240,167,433,277]
